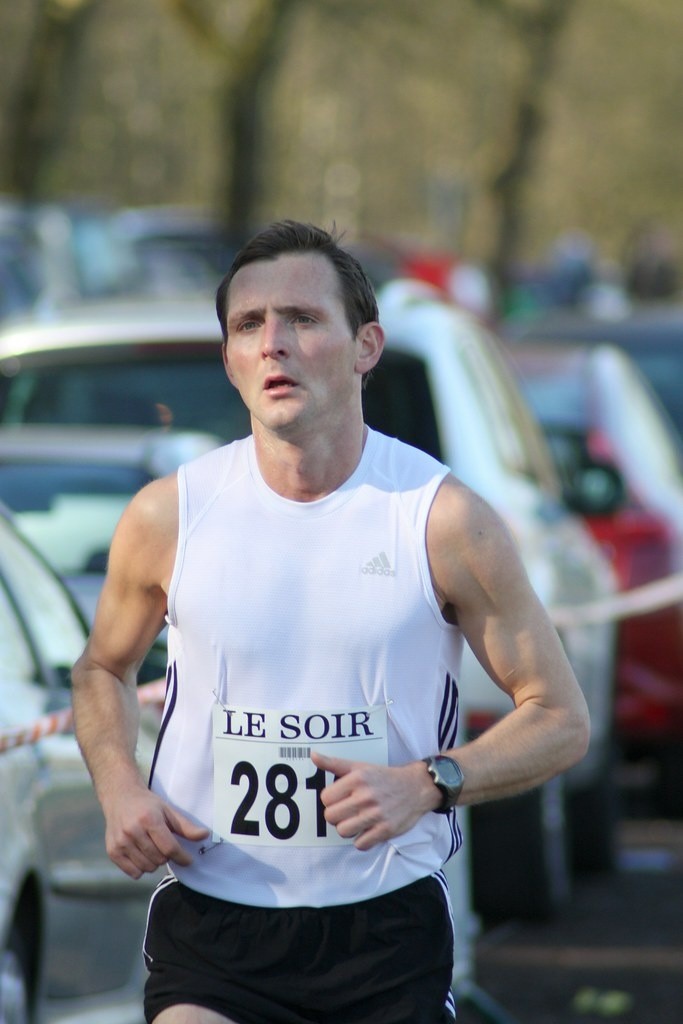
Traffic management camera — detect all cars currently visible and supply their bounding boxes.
[1,193,683,1024]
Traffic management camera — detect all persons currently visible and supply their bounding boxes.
[67,221,592,1024]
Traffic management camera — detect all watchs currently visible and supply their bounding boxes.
[421,754,464,815]
